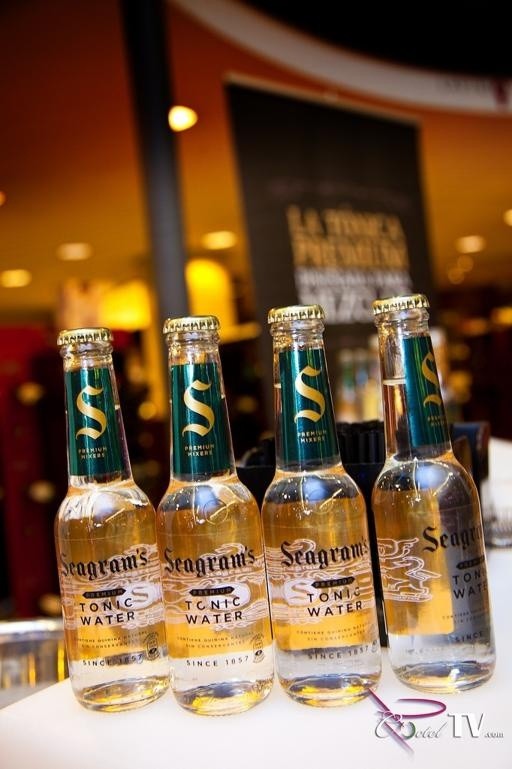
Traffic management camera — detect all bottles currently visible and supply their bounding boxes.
[51,329,168,715]
[365,297,497,695]
[259,304,383,710]
[155,314,276,719]
[327,346,381,426]
